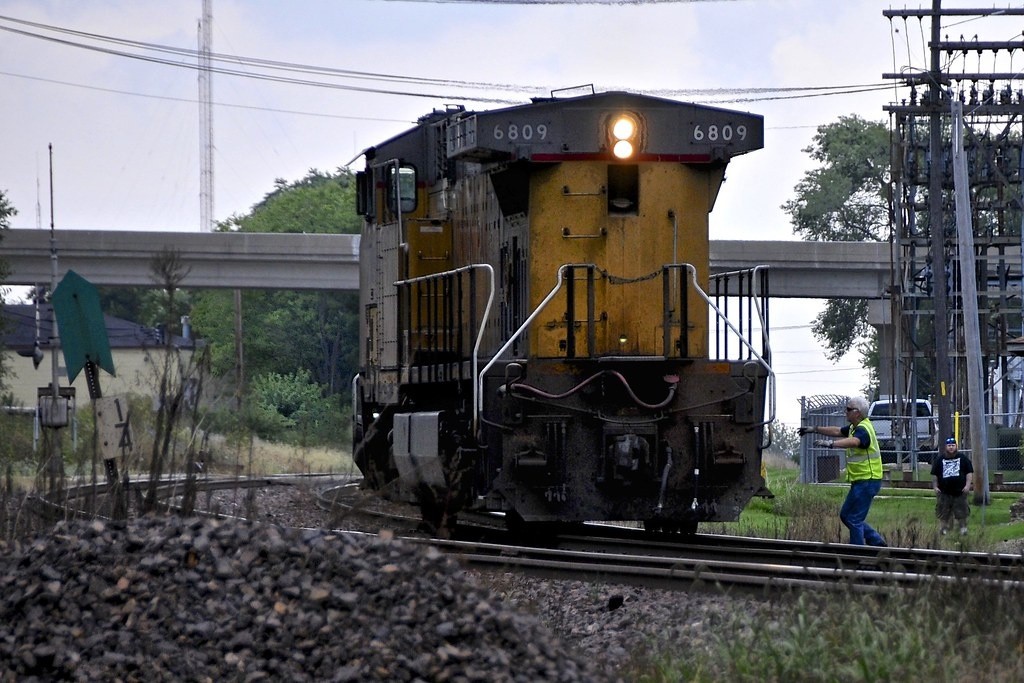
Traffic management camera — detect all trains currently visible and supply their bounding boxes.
[353,85,775,535]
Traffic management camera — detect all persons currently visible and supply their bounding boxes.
[930,438,974,535]
[798,396,888,548]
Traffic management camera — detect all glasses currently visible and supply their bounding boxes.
[946,437,956,444]
[846,407,857,411]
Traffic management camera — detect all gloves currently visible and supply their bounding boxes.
[812,439,834,449]
[796,425,818,436]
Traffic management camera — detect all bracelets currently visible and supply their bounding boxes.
[829,440,834,449]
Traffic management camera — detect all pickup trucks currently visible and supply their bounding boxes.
[866,398,938,457]
